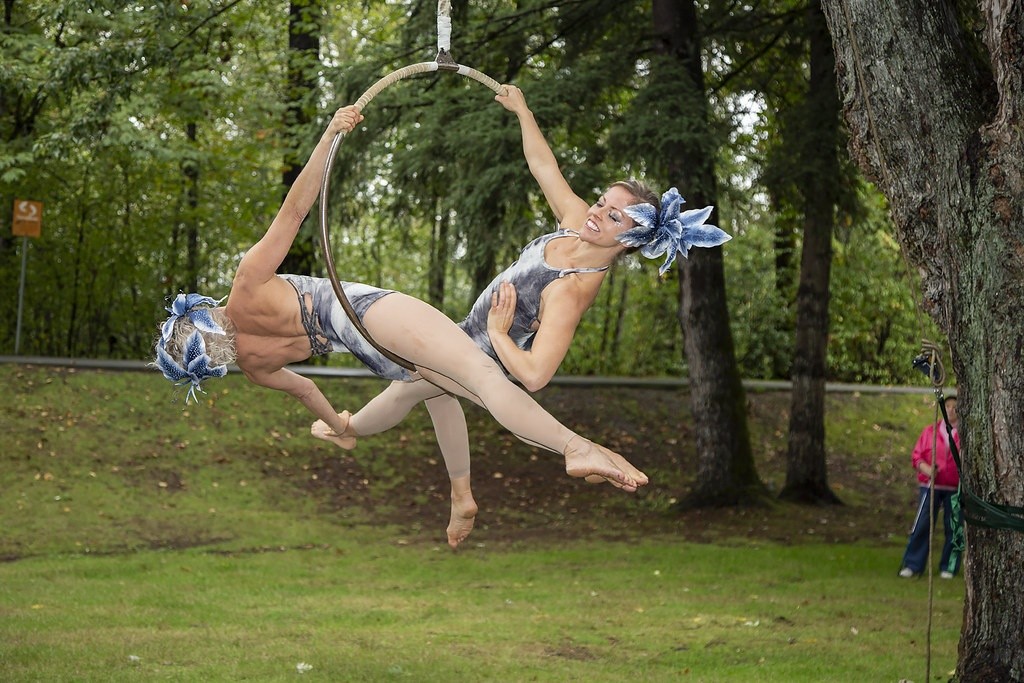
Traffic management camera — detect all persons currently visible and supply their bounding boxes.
[309,84,732,547]
[153,105,649,494]
[900,396,963,579]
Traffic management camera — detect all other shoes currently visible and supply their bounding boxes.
[941,570,953,579]
[900,566,913,578]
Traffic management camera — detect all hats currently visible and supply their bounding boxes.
[943,393,958,402]
